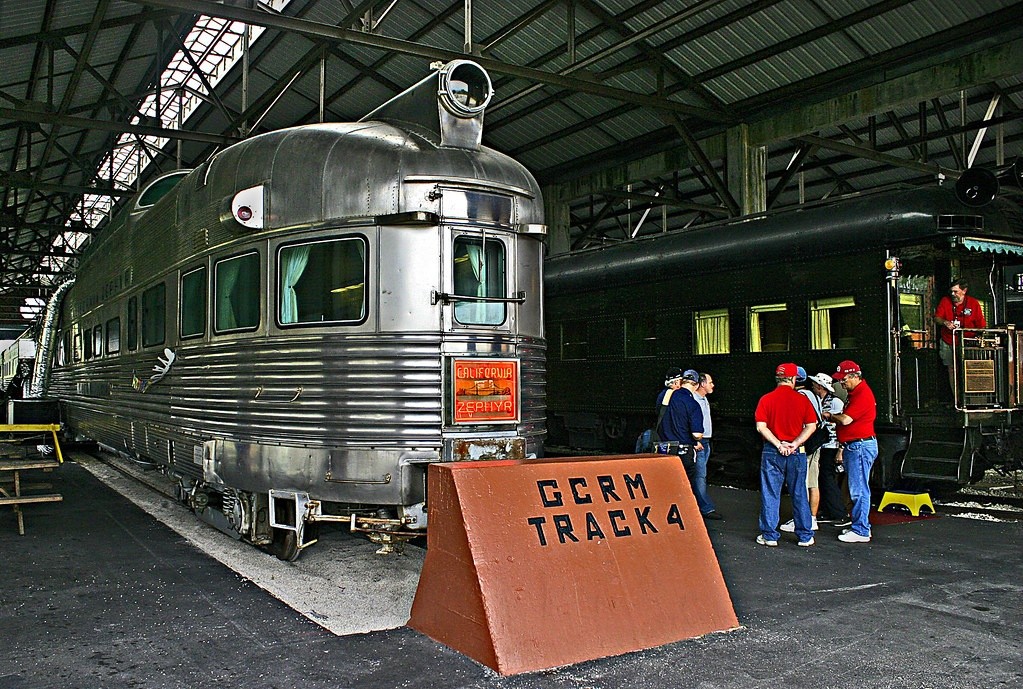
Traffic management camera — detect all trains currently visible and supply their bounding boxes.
[545,164,1023,500]
[0,56,548,561]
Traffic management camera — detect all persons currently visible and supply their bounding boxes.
[656,367,683,414]
[755,363,818,546]
[656,369,704,483]
[933,278,986,408]
[806,372,853,527]
[821,361,878,542]
[780,367,822,532]
[693,373,723,519]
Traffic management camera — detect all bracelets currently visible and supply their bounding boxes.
[839,445,844,449]
[830,414,832,421]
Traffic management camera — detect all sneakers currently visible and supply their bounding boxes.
[780,515,818,532]
[838,529,871,543]
[816,513,836,523]
[834,517,853,527]
[756,535,777,546]
[798,536,815,547]
[843,527,872,537]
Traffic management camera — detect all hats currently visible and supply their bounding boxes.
[664,367,682,384]
[808,372,835,393]
[832,360,860,379]
[682,370,699,383]
[775,363,800,377]
[794,366,806,382]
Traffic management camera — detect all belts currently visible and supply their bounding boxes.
[842,436,875,448]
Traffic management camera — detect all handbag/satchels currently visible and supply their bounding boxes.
[803,424,830,456]
[636,430,658,454]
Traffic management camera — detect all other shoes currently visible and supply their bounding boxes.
[702,509,723,520]
